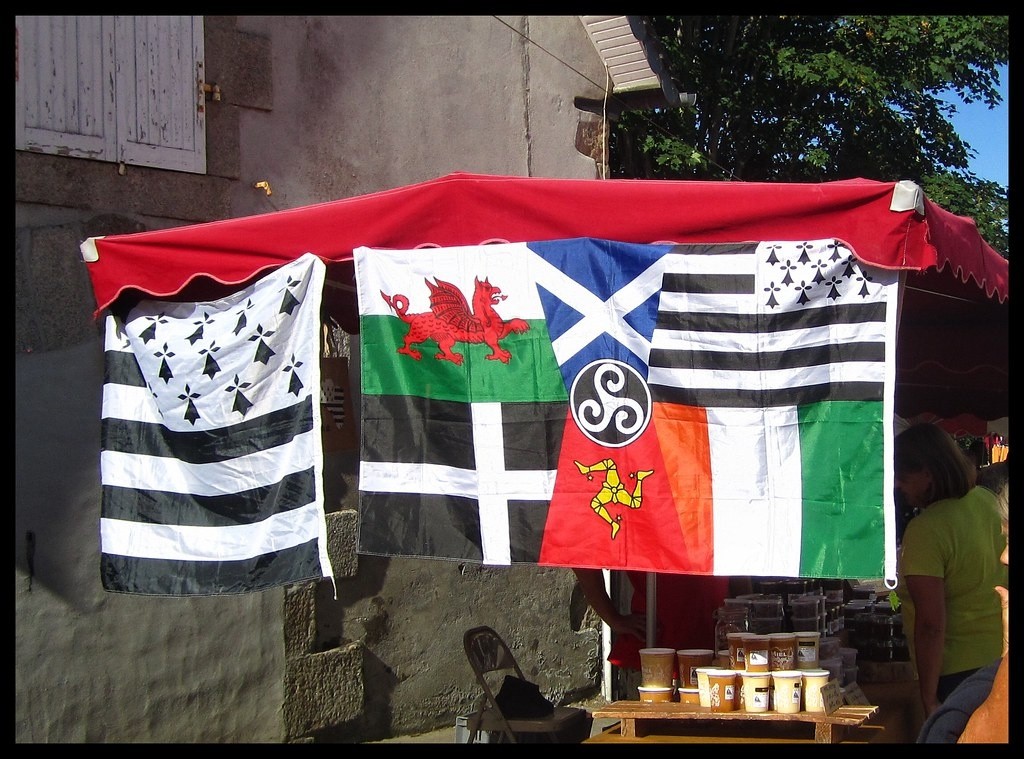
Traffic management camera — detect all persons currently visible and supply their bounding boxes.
[572,567,729,699]
[895,423,1009,715]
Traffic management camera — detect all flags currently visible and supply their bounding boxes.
[101,254,332,595]
[354,239,898,581]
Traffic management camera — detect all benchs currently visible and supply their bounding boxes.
[591,699,880,744]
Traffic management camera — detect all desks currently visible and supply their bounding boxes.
[581,678,926,744]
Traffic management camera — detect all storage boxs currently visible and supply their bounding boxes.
[455,711,518,744]
[723,576,909,687]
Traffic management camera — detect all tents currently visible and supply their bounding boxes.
[81,170,1009,701]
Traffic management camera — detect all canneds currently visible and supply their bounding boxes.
[758,578,846,635]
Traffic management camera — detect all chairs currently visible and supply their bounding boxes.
[463,625,587,744]
